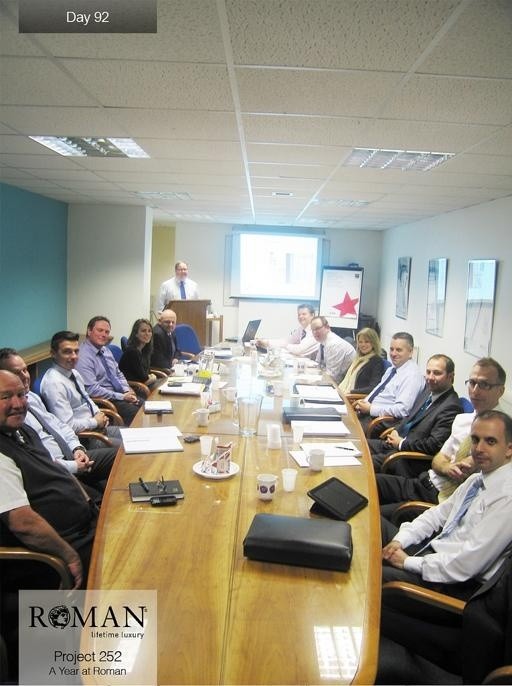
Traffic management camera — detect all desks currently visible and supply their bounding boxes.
[80,339,381,686]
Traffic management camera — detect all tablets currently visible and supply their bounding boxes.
[306,476,368,521]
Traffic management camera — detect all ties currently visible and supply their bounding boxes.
[401,397,432,437]
[99,350,125,393]
[438,437,470,502]
[71,374,94,417]
[27,405,74,460]
[180,281,186,299]
[411,476,483,557]
[319,344,324,363]
[368,368,396,402]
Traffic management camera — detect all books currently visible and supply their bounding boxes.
[283,382,363,467]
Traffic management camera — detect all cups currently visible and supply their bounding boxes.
[306,449,324,472]
[256,474,279,501]
[174,340,306,455]
[281,469,298,492]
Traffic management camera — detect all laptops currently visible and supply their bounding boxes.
[224,319,262,343]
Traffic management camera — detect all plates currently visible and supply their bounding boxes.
[192,460,240,479]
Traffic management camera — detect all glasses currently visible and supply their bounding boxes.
[465,380,500,390]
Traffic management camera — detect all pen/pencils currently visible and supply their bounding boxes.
[336,446,354,450]
[139,477,149,493]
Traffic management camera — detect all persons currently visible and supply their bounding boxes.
[376,354,507,520]
[310,316,356,384]
[338,327,386,403]
[352,331,426,439]
[157,261,201,312]
[0,308,185,604]
[371,553,511,683]
[380,410,512,603]
[367,354,463,479]
[280,304,320,361]
